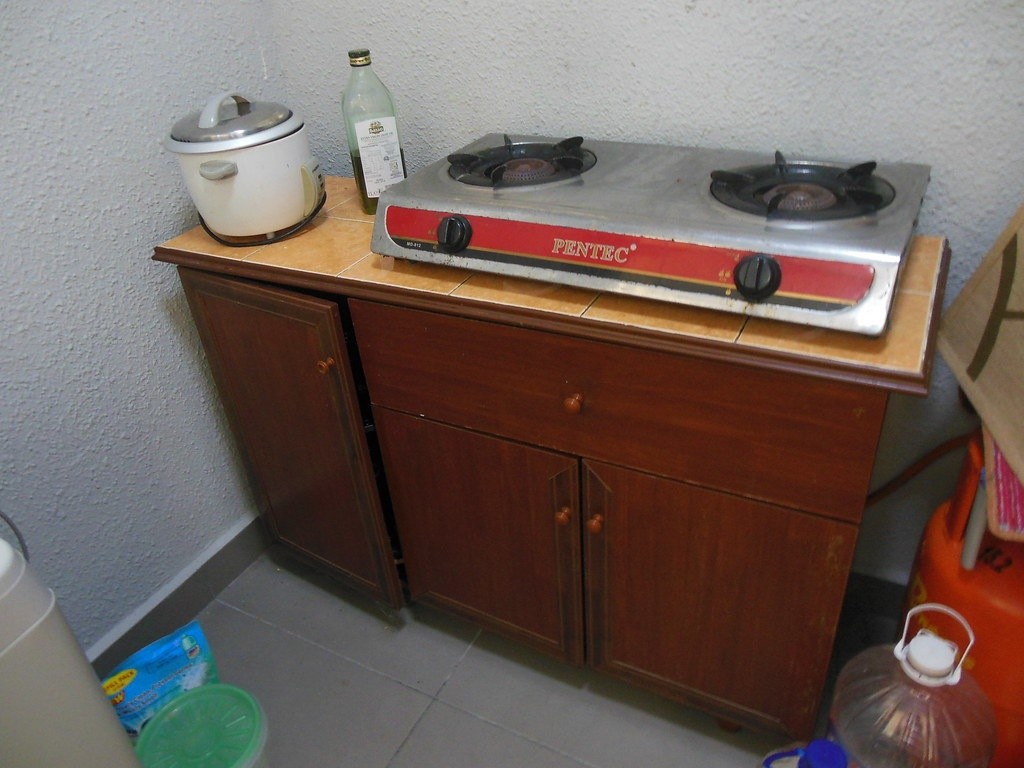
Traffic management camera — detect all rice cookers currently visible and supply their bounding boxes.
[164,91,325,244]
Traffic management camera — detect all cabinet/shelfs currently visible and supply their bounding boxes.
[150,175,953,740]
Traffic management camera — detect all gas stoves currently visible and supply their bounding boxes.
[370,135,931,335]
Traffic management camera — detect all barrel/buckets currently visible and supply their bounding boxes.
[136,682,270,768]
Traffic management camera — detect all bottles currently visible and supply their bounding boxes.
[760,738,847,768]
[341,49,407,215]
[825,603,994,768]
